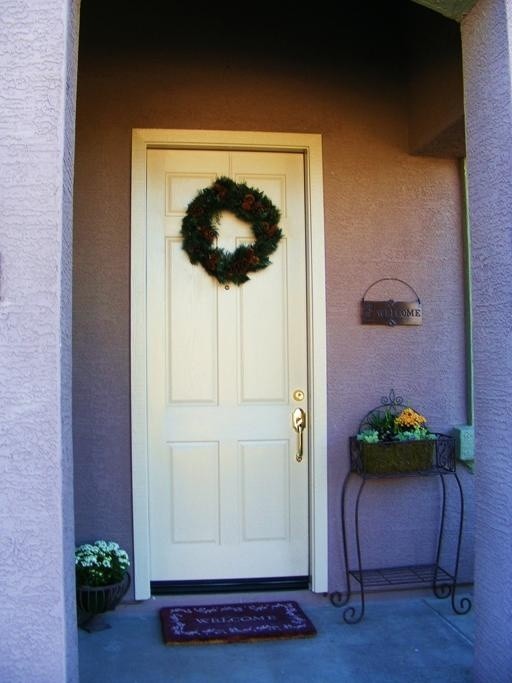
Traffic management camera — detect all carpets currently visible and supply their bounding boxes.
[158,598,318,645]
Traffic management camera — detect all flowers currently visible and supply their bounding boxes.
[73,538,131,576]
[354,407,436,440]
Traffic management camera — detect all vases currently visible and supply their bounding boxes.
[346,438,438,473]
[75,573,131,633]
